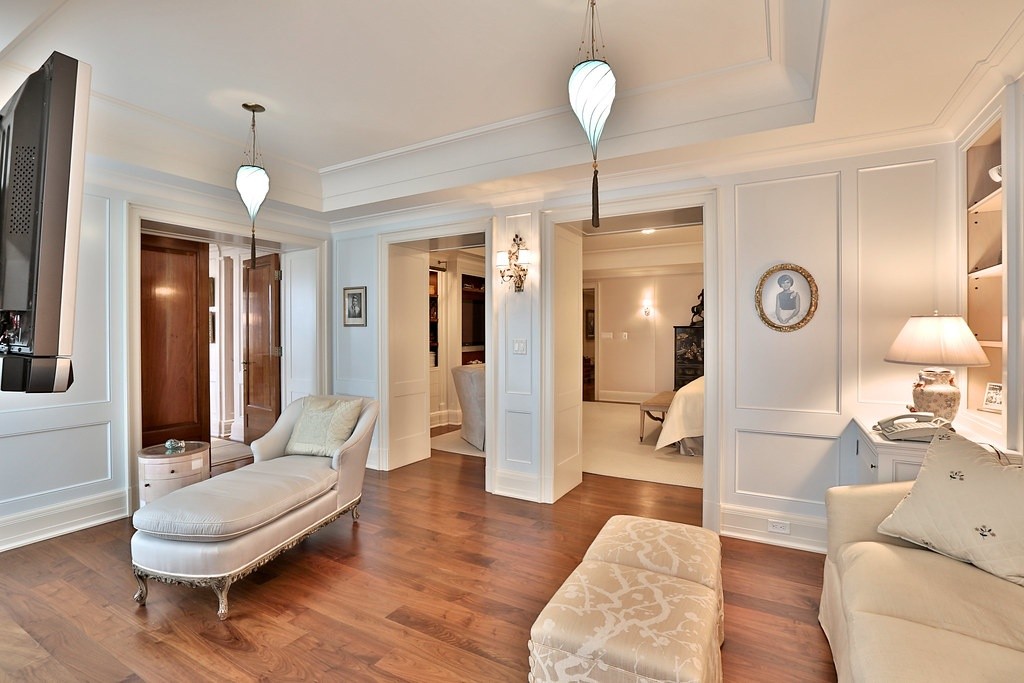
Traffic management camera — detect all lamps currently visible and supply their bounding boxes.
[883,310,991,425]
[235,103,270,268]
[496,234,531,293]
[568,0,617,228]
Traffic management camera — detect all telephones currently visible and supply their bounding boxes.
[877,412,954,443]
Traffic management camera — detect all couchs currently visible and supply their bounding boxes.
[818,478,1024,683]
[131,395,380,621]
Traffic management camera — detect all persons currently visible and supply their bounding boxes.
[776,275,800,323]
[349,295,361,317]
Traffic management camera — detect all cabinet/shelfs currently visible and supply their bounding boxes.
[852,416,1024,487]
[674,321,704,392]
[461,273,486,366]
[428,270,440,369]
[963,109,1007,427]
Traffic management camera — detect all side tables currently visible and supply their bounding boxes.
[136,441,209,511]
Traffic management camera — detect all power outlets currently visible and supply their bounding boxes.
[768,519,791,535]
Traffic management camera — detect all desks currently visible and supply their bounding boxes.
[451,363,485,452]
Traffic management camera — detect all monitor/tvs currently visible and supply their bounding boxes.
[0,50,92,393]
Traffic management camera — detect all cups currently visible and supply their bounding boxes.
[430,351,436,367]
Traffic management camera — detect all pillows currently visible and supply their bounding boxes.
[875,427,1024,588]
[284,393,364,458]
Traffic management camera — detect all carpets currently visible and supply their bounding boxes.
[211,435,254,466]
[430,400,702,490]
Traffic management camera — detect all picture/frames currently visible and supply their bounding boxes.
[585,309,595,341]
[343,286,367,327]
[755,263,819,333]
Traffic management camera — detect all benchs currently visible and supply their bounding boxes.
[528,515,725,683]
[640,390,676,443]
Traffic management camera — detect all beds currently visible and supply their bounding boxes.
[655,375,704,458]
